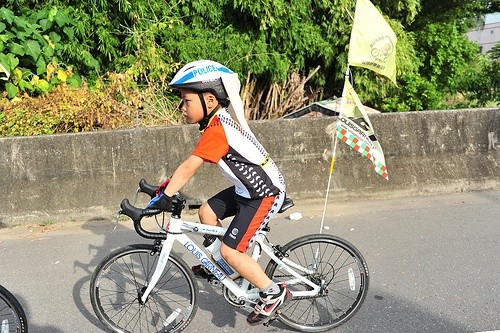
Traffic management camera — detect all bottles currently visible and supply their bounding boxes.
[202,234,240,280]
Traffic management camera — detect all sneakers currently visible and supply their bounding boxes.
[247,283,293,326]
[193,264,217,279]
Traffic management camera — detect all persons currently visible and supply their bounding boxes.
[145,60,293,326]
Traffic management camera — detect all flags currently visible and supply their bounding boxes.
[348,0,398,88]
[336,78,389,181]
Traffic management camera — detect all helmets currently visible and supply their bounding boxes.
[168,59,240,99]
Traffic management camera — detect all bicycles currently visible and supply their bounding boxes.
[90,178,370,333]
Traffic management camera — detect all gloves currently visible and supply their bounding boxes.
[156,179,170,196]
[144,188,175,218]
